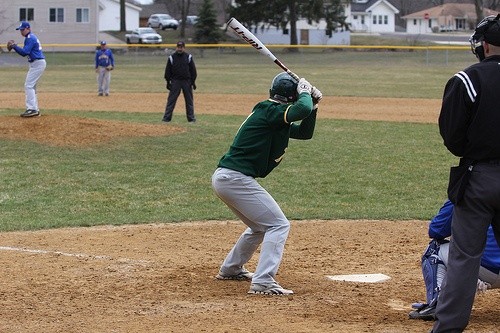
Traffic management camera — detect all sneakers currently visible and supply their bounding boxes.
[248,282,294,295]
[20,109,40,117]
[410,304,437,320]
[216,265,254,280]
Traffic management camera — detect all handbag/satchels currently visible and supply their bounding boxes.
[448,154,472,206]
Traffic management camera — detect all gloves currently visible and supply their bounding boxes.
[297,78,312,94]
[167,81,172,90]
[311,86,323,103]
[191,82,196,90]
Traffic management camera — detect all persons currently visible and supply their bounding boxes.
[408,200,500,321]
[94,40,114,97]
[429,14,500,333]
[6,22,46,117]
[212,72,322,295]
[162,41,198,122]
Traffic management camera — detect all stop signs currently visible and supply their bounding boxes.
[425,13,429,19]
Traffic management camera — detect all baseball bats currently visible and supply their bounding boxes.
[226,18,320,102]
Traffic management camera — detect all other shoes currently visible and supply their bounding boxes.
[99,93,103,96]
[105,93,110,96]
[188,116,196,121]
[162,117,171,121]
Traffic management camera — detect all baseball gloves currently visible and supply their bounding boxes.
[7,40,15,51]
[105,66,113,71]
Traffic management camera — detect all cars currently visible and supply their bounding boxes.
[178,15,200,25]
[147,14,178,30]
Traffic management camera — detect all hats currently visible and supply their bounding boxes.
[101,41,106,44]
[177,41,184,46]
[16,21,30,31]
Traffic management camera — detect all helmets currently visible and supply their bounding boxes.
[469,14,500,63]
[269,71,300,104]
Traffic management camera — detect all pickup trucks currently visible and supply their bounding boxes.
[125,28,162,48]
